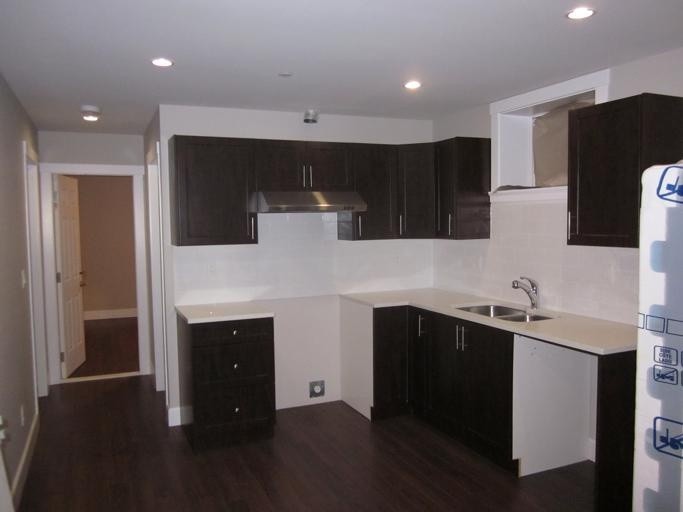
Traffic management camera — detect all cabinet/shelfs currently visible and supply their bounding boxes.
[427,310,590,476]
[435,137,491,239]
[390,142,435,239]
[177,312,275,456]
[254,139,353,191]
[339,297,428,423]
[337,142,397,241]
[567,93,683,247]
[168,135,258,246]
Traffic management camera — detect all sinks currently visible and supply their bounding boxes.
[497,313,553,323]
[454,305,525,324]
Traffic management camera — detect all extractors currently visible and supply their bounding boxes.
[254,190,367,213]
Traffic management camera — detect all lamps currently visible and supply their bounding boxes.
[80,105,100,122]
[304,110,318,123]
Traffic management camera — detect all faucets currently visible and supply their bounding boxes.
[512,274,541,309]
